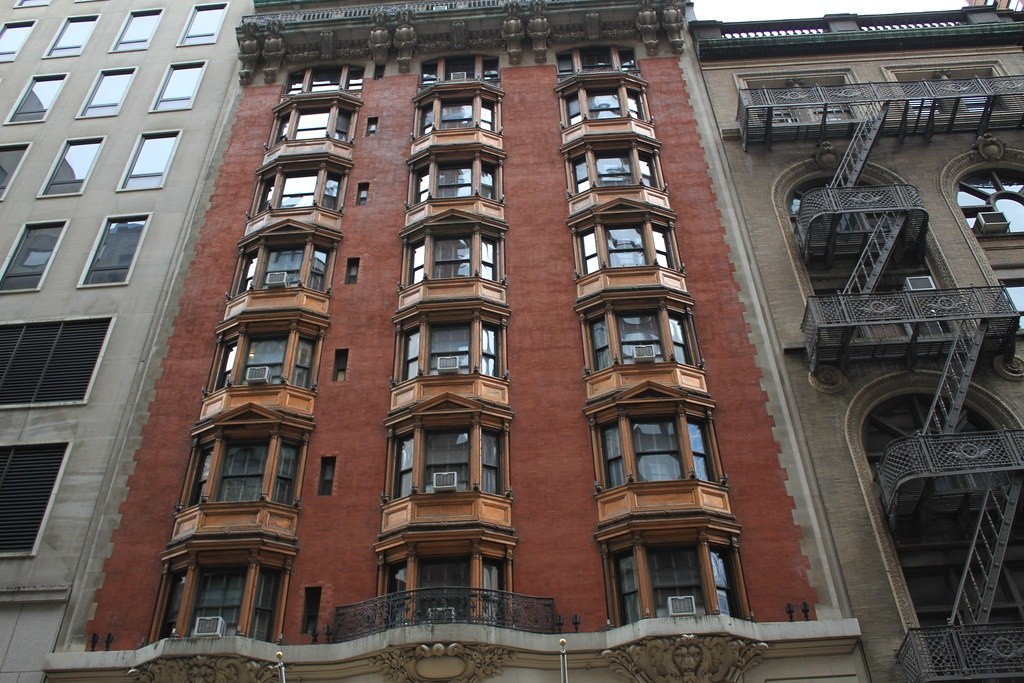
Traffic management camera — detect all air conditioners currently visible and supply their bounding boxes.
[667,596,696,616]
[450,71,467,82]
[902,275,937,293]
[193,616,227,637]
[632,344,657,363]
[976,209,1013,233]
[246,366,272,384]
[265,271,290,289]
[432,470,458,492]
[428,606,458,623]
[436,355,460,374]
